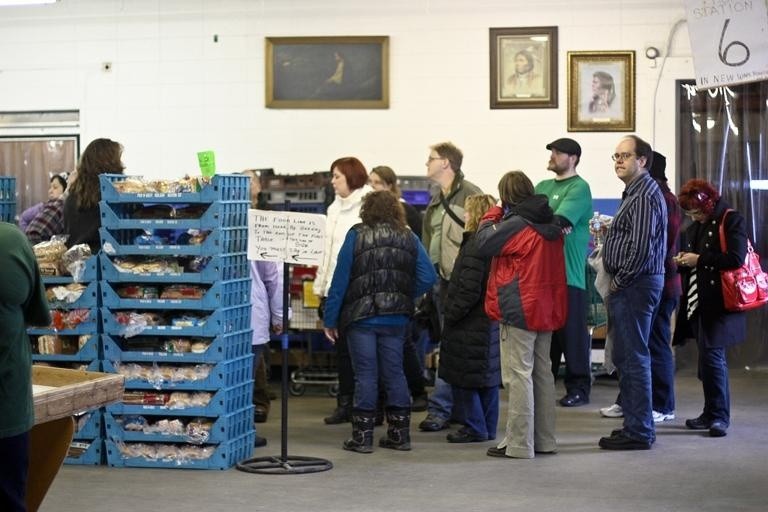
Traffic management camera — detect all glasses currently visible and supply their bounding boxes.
[612,153,636,161]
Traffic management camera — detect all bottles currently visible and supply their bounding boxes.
[590,210,604,248]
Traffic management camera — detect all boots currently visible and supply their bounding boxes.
[344,409,374,453]
[379,407,412,450]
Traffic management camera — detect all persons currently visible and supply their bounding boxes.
[246,172,287,446]
[438,194,505,441]
[323,190,436,452]
[672,178,749,434]
[600,135,670,451]
[368,165,429,411]
[419,142,483,430]
[313,156,382,426]
[475,172,567,460]
[503,49,546,96]
[601,148,683,422]
[581,72,620,119]
[19,137,126,255]
[0,215,52,512]
[533,138,594,406]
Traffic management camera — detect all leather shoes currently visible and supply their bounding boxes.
[560,394,589,406]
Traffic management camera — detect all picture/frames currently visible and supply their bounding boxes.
[489,25,558,109]
[265,35,390,110]
[566,50,636,132]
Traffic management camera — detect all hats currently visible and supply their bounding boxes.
[547,139,580,155]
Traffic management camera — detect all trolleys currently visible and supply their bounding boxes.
[285,264,343,395]
[584,260,618,388]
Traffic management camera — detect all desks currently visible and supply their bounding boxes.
[26,362,125,512]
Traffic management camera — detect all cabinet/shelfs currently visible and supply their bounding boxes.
[261,180,431,345]
[28,258,97,461]
[0,176,17,221]
[98,171,255,469]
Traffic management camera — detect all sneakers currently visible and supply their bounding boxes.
[599,404,727,449]
[419,414,506,457]
[325,407,350,424]
[255,405,268,422]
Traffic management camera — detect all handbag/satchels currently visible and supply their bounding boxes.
[721,246,768,312]
